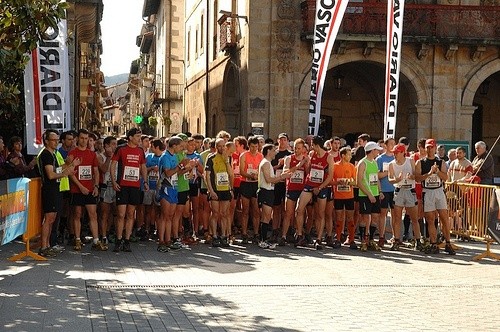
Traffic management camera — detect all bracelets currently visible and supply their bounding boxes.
[379,191,382,193]
[143,182,148,184]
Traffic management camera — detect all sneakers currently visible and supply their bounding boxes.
[50,243,66,253]
[173,238,190,248]
[113,239,131,251]
[38,247,63,257]
[73,239,82,251]
[445,244,456,255]
[158,242,181,253]
[92,241,108,250]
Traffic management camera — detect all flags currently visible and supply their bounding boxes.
[383,0,404,144]
[22,0,70,154]
[308,0,349,137]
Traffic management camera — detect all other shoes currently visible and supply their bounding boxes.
[388,236,431,252]
[314,239,322,250]
[130,228,159,242]
[292,233,313,246]
[258,240,279,248]
[181,232,238,247]
[325,232,385,251]
[241,234,249,244]
[252,234,261,244]
[425,243,439,255]
[278,237,285,246]
[67,231,118,245]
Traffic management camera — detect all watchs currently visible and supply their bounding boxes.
[435,168,439,173]
[318,186,321,191]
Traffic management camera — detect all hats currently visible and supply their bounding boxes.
[278,133,288,139]
[391,145,405,152]
[417,139,426,146]
[425,139,436,147]
[399,137,410,145]
[364,141,383,151]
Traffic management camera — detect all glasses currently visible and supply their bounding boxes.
[48,138,60,142]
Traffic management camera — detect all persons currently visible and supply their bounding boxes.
[329,147,358,250]
[388,143,426,252]
[256,144,292,249]
[110,127,149,253]
[0,127,473,256]
[415,138,456,255]
[471,141,494,234]
